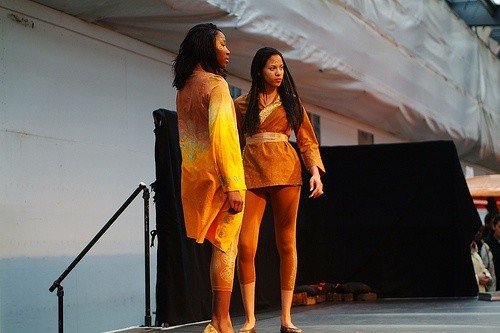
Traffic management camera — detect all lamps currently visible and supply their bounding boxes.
[444,0,500,60]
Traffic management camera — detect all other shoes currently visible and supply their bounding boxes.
[238,325,256,333]
[202,323,219,333]
[280,324,302,333]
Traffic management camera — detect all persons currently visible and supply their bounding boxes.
[171,23,247,333]
[234,47,326,333]
[470,213,500,293]
